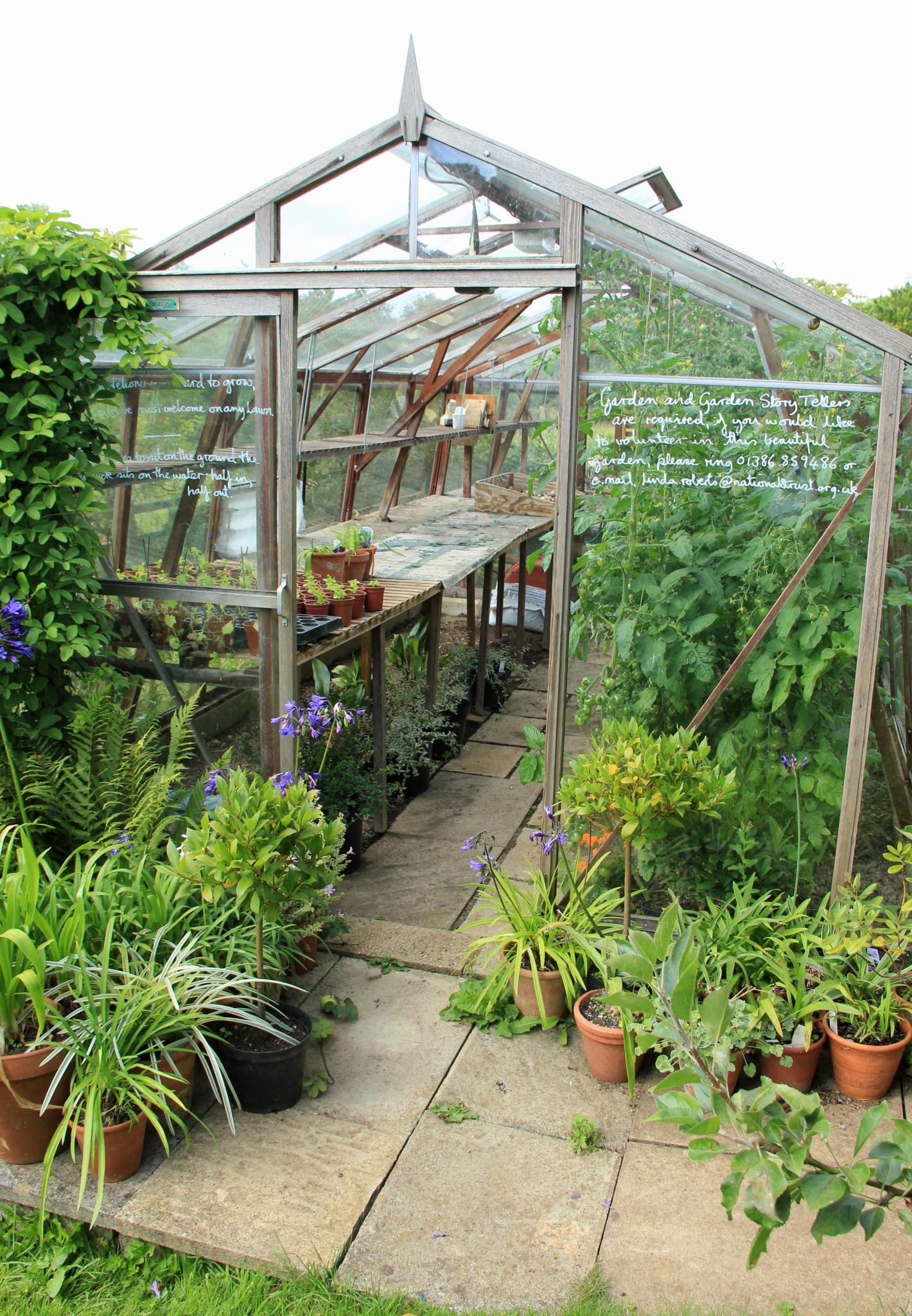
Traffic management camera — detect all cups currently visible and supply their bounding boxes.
[452,415,465,429]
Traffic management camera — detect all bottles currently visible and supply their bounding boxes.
[445,396,457,426]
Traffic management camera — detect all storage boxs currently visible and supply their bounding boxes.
[474,472,586,516]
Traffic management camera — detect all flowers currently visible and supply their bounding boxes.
[724,750,813,992]
[199,691,363,925]
[449,800,613,1031]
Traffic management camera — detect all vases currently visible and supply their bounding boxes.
[288,931,321,974]
[573,983,646,1084]
[502,939,565,1023]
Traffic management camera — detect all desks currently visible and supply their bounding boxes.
[110,486,554,834]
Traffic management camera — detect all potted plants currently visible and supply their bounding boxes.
[299,613,536,875]
[551,714,912,1100]
[100,520,387,655]
[1,764,339,1253]
